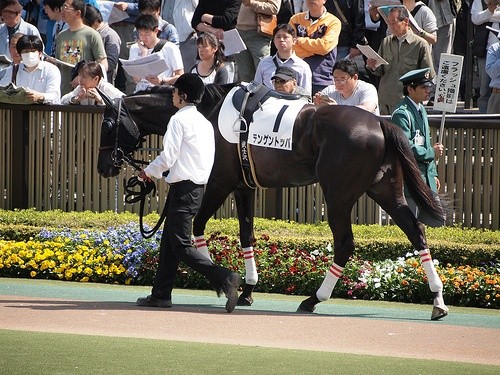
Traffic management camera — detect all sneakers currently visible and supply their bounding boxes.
[222,272,240,312]
[137,296,172,308]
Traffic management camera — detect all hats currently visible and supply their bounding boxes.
[399,68,435,87]
[271,66,296,81]
[168,73,204,103]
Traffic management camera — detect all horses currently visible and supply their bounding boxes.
[95,81,459,321]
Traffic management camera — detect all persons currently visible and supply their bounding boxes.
[391,66,445,227]
[136,73,242,312]
[0,0,500,214]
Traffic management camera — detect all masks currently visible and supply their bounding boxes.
[20,51,40,67]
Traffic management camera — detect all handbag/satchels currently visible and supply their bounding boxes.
[256,13,277,37]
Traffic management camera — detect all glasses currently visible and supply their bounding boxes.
[60,5,74,11]
[272,80,284,85]
